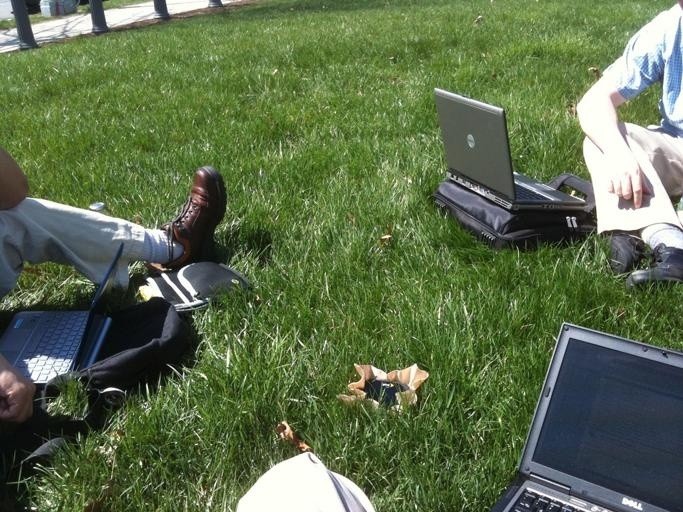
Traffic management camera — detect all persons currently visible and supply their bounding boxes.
[1,150,227,425]
[578,0,682,288]
[237,454,373,512]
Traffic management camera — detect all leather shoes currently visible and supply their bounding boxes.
[160,167,226,267]
[626,244,683,288]
[609,234,644,275]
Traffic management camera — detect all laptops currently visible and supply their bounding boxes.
[0,240,130,386]
[488,321,683,512]
[432,86,588,212]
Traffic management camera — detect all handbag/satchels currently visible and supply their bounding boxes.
[429,179,597,250]
[7,296,182,436]
